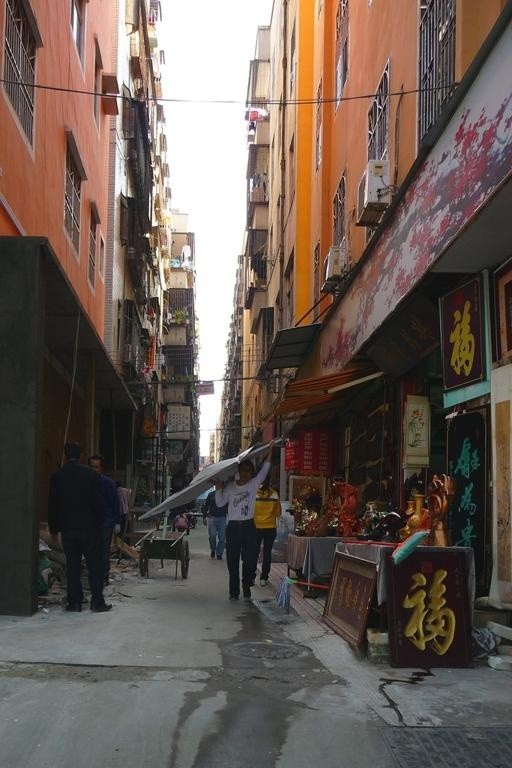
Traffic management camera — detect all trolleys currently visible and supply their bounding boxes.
[133,526,189,580]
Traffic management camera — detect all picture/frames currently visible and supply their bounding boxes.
[490,257,512,370]
[444,405,491,598]
[440,268,488,392]
[383,548,478,668]
[322,552,379,653]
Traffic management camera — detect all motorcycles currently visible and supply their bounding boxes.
[189,509,198,528]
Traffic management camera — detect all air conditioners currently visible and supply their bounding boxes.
[321,246,346,292]
[355,159,389,229]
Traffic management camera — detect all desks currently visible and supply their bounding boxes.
[287,533,359,591]
[334,541,476,632]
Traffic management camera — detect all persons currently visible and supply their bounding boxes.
[173,511,190,534]
[85,455,124,589]
[208,443,275,602]
[49,441,113,611]
[251,472,282,585]
[203,487,228,559]
[115,480,131,539]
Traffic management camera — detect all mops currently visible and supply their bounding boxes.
[274,574,331,615]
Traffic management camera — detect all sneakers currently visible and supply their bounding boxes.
[260,580,265,586]
[65,605,83,611]
[91,603,112,610]
[211,550,215,556]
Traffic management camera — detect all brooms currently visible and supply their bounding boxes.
[342,528,429,565]
